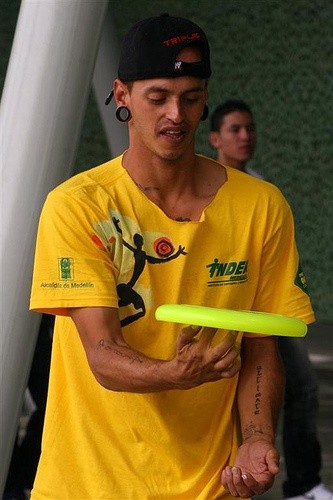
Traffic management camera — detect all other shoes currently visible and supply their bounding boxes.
[288,483,333,500]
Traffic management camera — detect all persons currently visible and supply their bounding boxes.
[207,99,333,500]
[27,11,315,500]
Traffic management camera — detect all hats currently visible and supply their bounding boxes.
[117,13,212,82]
